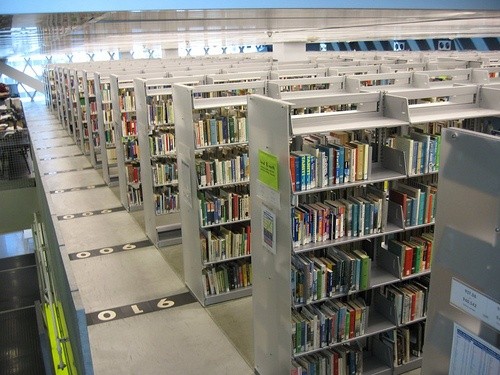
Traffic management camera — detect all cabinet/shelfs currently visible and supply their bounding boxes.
[45,51,500,375]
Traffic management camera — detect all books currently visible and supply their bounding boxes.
[202,259,251,298]
[119,88,143,205]
[100,83,115,143]
[195,115,247,147]
[87,80,99,146]
[150,95,181,213]
[194,89,254,98]
[201,224,251,263]
[293,103,357,115]
[292,172,436,249]
[281,84,329,90]
[44,68,88,148]
[198,183,250,226]
[360,79,394,86]
[291,318,428,375]
[195,143,248,187]
[292,224,435,306]
[290,118,500,192]
[290,274,431,354]
